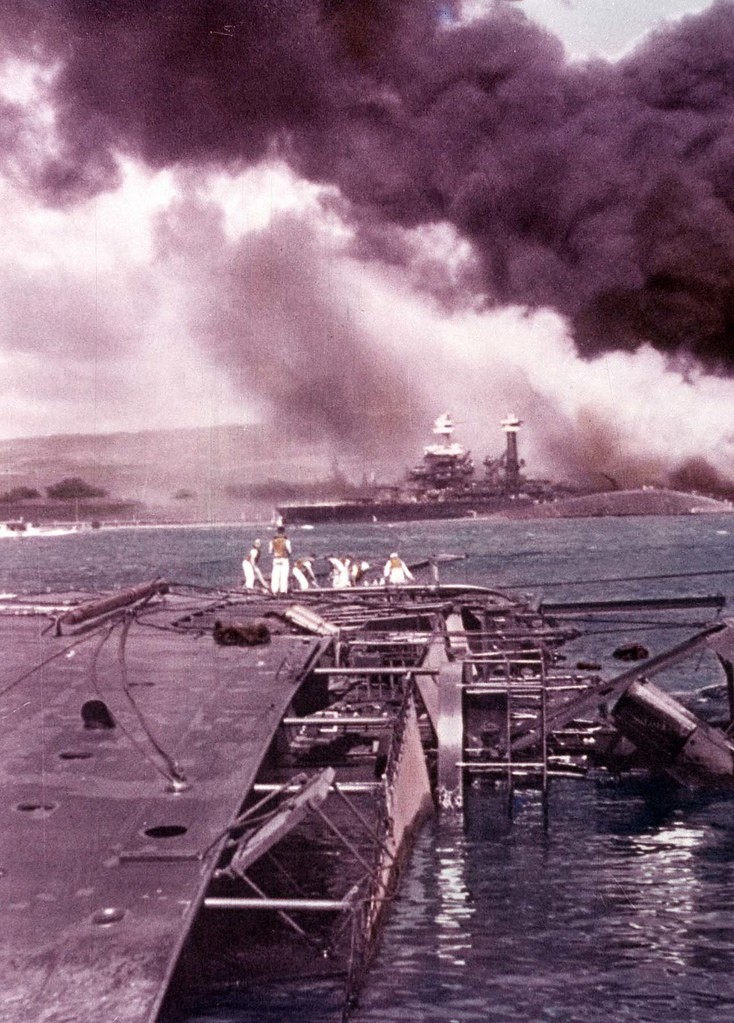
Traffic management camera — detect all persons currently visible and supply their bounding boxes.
[384,551,417,586]
[292,553,318,589]
[269,525,292,593]
[325,552,375,589]
[242,539,261,590]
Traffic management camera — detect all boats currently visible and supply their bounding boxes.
[273,411,623,526]
